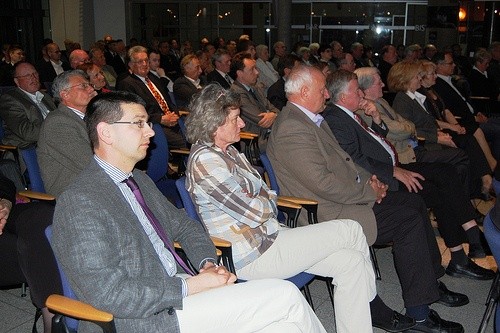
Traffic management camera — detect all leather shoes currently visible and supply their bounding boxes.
[372,310,416,332]
[405,309,465,333]
[467,242,494,257]
[434,280,469,307]
[446,255,496,280]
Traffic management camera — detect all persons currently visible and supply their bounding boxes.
[267,65,470,333]
[0,35,500,225]
[183,83,417,333]
[322,70,496,280]
[36,69,98,196]
[119,46,186,148]
[51,91,328,333]
[0,171,17,234]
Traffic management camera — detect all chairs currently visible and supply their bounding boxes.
[0,109,380,333]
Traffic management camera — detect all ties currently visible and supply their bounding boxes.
[226,75,232,85]
[121,176,196,277]
[353,112,400,166]
[145,78,169,114]
[249,88,259,102]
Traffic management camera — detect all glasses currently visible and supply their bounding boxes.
[67,82,90,89]
[442,62,454,65]
[110,120,153,130]
[17,73,38,78]
